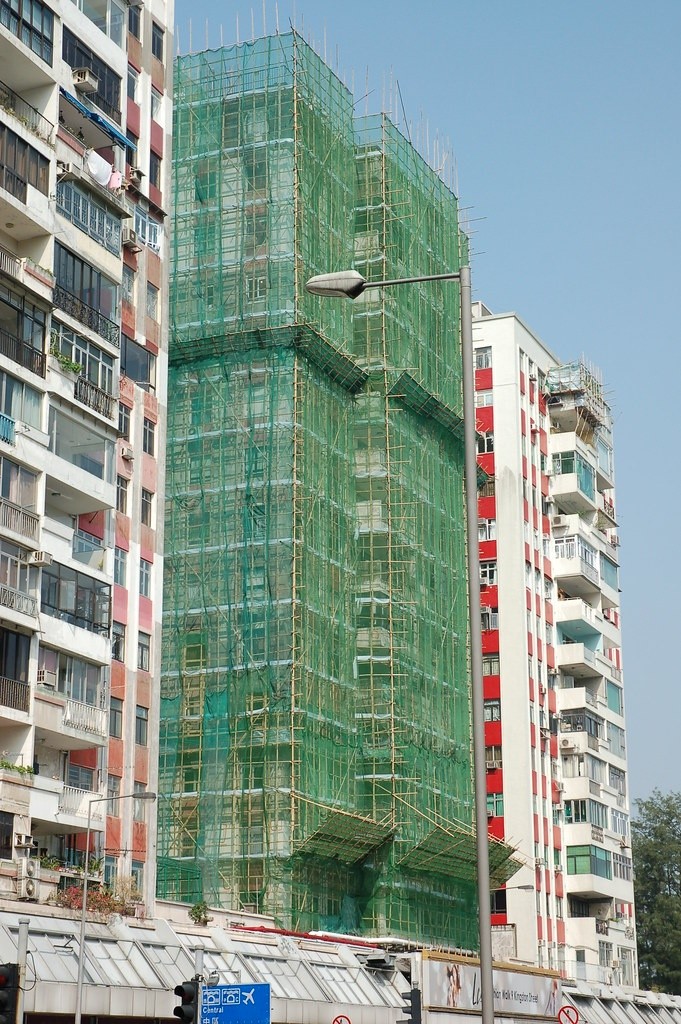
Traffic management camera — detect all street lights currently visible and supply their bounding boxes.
[73,791,160,1024]
[307,265,502,1024]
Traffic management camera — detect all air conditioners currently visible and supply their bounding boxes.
[555,864,563,871]
[536,857,544,864]
[558,737,575,748]
[17,857,41,879]
[612,535,619,545]
[612,960,622,969]
[486,760,497,769]
[17,877,40,901]
[481,606,492,613]
[121,447,135,461]
[15,835,34,848]
[131,171,142,183]
[37,670,56,687]
[74,70,98,93]
[122,228,137,247]
[550,514,567,528]
[531,424,538,431]
[530,373,536,380]
[57,163,81,181]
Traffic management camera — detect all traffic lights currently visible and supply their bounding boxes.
[0,963,20,1024]
[395,988,422,1024]
[174,980,200,1024]
